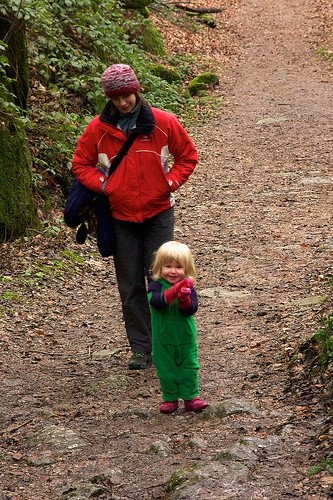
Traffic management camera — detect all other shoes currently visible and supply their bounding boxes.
[159,400,178,413]
[183,396,209,411]
[127,352,152,370]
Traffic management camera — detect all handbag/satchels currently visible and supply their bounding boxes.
[95,194,113,257]
[64,177,104,244]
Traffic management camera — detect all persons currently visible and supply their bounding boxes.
[146,240,210,413]
[70,64,199,370]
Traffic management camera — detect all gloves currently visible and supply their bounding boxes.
[177,286,192,309]
[163,276,196,304]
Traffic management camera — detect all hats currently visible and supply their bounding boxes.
[99,64,139,98]
